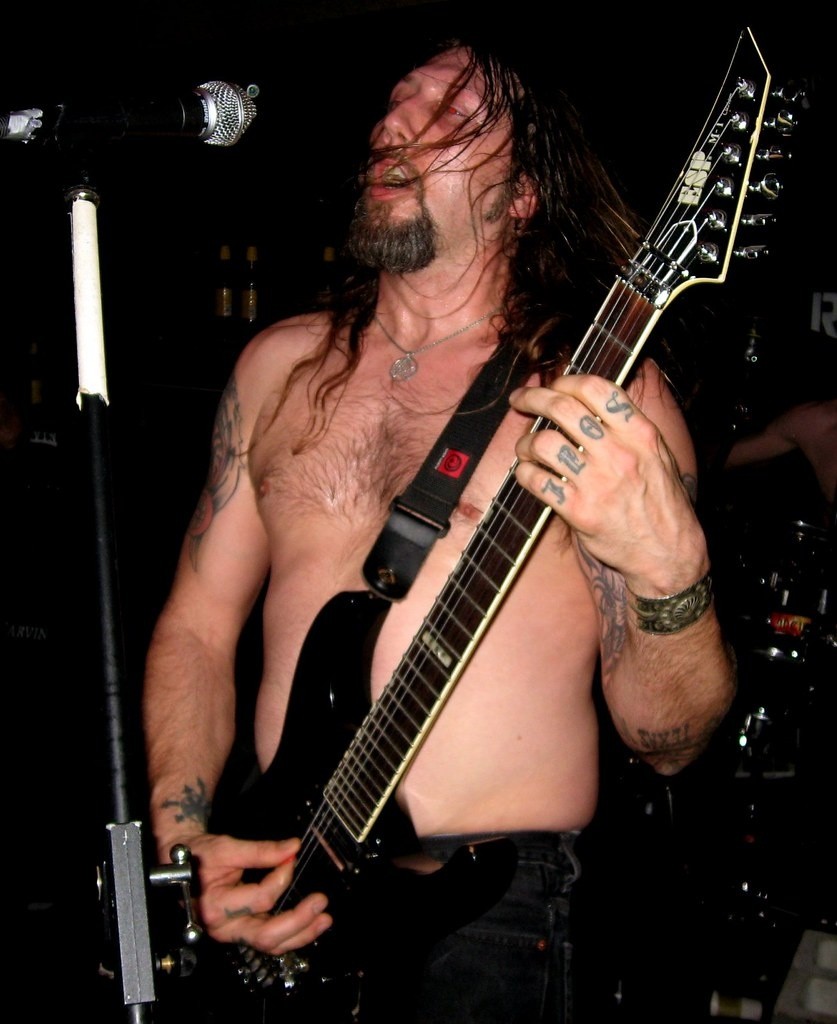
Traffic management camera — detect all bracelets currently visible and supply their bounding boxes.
[624,566,714,633]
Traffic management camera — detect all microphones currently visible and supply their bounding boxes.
[0,81,259,146]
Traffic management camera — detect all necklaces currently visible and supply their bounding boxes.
[373,308,501,380]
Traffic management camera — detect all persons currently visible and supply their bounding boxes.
[0,393,60,449]
[142,31,737,1024]
[723,397,837,507]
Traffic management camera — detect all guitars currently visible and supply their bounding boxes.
[169,12,808,1024]
